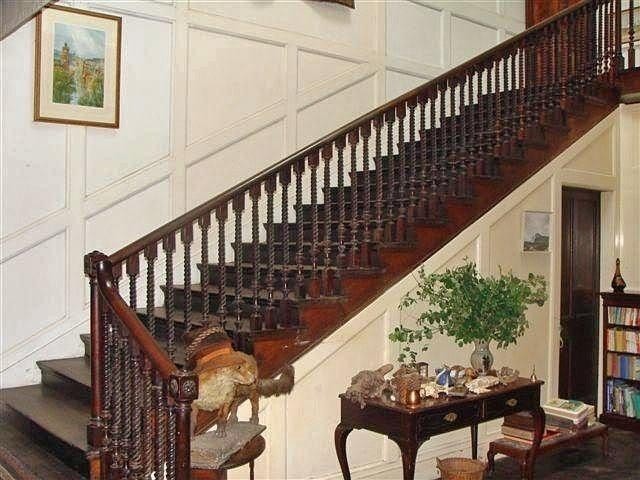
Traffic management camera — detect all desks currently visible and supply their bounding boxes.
[487,422,610,480]
[334,375,546,480]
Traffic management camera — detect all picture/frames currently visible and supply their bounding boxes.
[33,3,123,128]
[520,210,553,255]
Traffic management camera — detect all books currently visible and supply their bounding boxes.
[499,303,640,445]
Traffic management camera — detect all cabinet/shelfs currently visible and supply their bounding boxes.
[599,292,640,433]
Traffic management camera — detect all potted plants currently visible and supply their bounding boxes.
[387,254,549,375]
